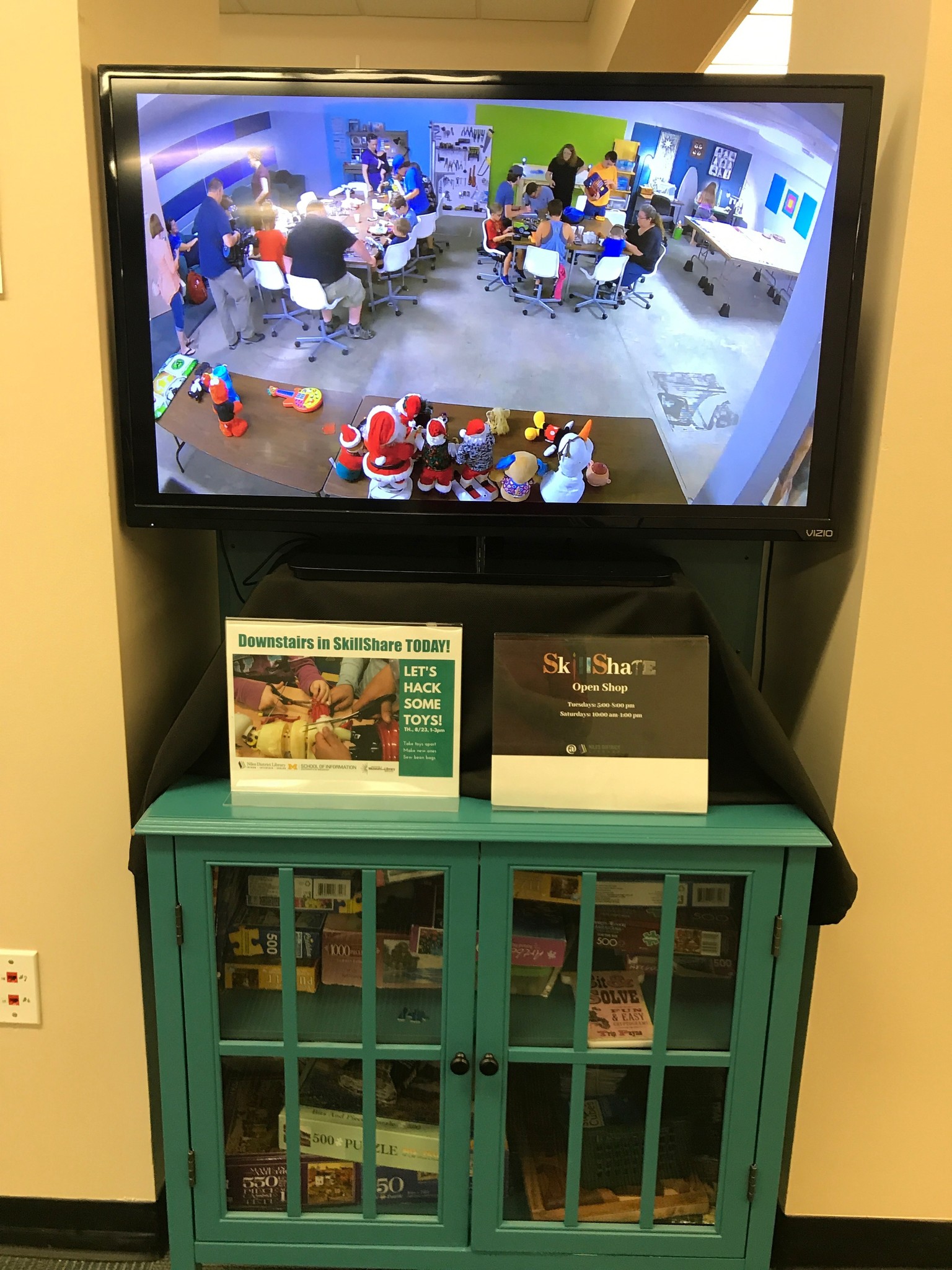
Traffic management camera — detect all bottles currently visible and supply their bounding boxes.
[213,366,240,403]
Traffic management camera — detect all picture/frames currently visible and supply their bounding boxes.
[706,145,739,181]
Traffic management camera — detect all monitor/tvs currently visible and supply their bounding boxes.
[95,63,887,589]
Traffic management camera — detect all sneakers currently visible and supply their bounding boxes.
[241,332,265,344]
[595,284,614,294]
[229,332,241,350]
[515,267,526,279]
[550,294,554,298]
[347,323,376,340]
[603,293,623,301]
[534,284,543,291]
[324,315,340,335]
[500,274,511,286]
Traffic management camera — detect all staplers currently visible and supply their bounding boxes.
[194,362,210,379]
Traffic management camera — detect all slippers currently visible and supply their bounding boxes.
[180,348,196,356]
[185,338,194,347]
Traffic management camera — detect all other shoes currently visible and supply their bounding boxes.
[569,257,578,265]
[690,241,698,245]
[427,248,436,261]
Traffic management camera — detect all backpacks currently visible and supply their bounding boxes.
[185,267,208,305]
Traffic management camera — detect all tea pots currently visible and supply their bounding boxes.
[586,460,611,486]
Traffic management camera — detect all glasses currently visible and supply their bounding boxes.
[636,214,649,220]
[531,191,537,199]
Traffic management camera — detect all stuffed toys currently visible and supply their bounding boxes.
[336,394,594,503]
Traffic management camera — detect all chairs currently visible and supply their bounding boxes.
[284,271,353,364]
[406,211,438,274]
[621,243,669,309]
[513,243,564,320]
[252,259,312,338]
[604,206,626,229]
[650,194,674,223]
[411,191,451,256]
[568,255,630,320]
[376,216,430,292]
[478,204,513,272]
[477,217,523,293]
[368,236,421,317]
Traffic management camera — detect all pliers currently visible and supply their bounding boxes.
[473,129,477,143]
[452,160,456,166]
[469,126,473,137]
[478,129,481,143]
[444,158,448,168]
[461,127,466,136]
[465,127,469,135]
[447,161,453,171]
[456,160,459,171]
[460,161,463,169]
[481,130,485,141]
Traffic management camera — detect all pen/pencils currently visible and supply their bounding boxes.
[343,253,352,255]
[344,255,354,257]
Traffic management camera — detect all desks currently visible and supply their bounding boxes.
[683,201,800,318]
[631,189,684,230]
[510,208,613,295]
[153,353,686,505]
[247,188,407,313]
[511,160,587,210]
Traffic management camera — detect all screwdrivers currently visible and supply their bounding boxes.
[439,180,442,193]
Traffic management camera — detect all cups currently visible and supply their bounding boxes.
[364,190,368,198]
[371,199,378,210]
[388,190,393,198]
[345,190,350,198]
[571,226,577,235]
[354,213,360,223]
[578,226,585,235]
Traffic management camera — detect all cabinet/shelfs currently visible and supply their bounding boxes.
[605,138,642,211]
[124,573,835,1270]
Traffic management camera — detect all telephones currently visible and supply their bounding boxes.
[583,172,608,198]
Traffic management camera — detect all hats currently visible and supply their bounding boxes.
[508,165,526,177]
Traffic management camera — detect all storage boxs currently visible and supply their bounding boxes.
[246,872,352,902]
[320,912,561,1000]
[473,927,566,968]
[594,875,731,911]
[278,1056,439,1173]
[358,1139,510,1206]
[224,901,322,957]
[224,1084,358,1210]
[638,183,653,195]
[222,958,320,995]
[592,905,730,960]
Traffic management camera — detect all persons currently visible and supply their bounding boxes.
[149,133,718,356]
[231,655,400,762]
